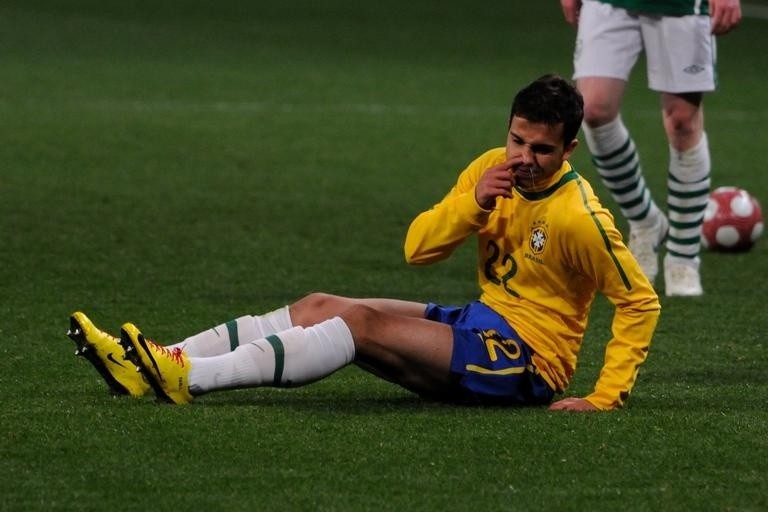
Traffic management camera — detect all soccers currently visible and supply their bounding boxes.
[701,186,764,253]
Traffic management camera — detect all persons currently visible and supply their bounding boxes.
[559,1,745,296]
[68,74,662,413]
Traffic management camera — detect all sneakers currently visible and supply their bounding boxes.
[66,312,151,396]
[117,323,194,404]
[627,211,670,283]
[663,252,704,297]
